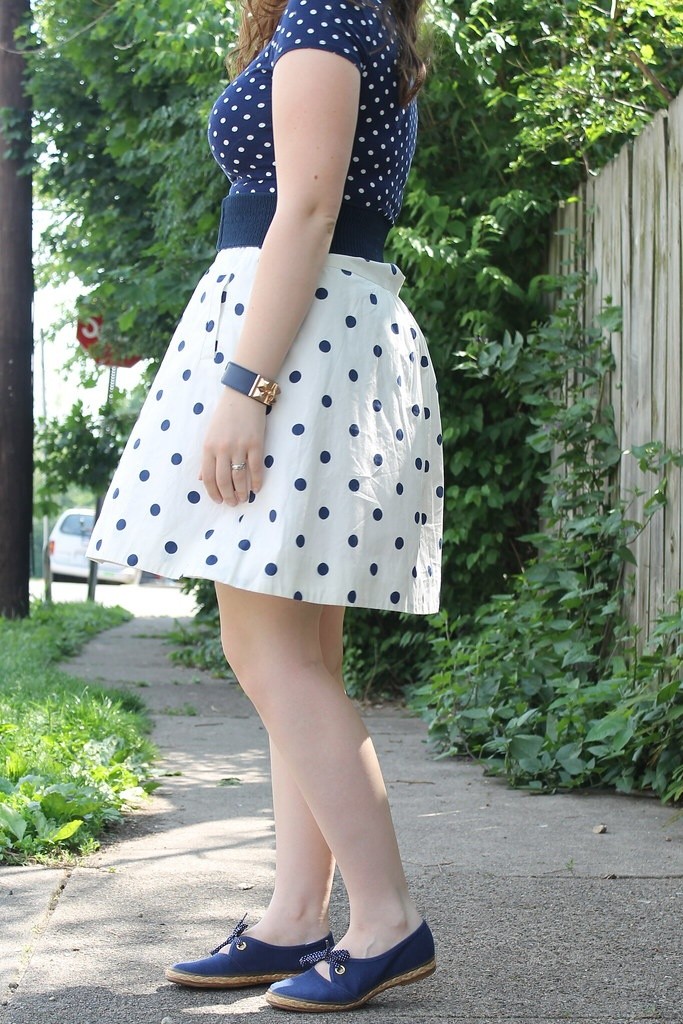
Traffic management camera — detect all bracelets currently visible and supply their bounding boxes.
[221,362,279,405]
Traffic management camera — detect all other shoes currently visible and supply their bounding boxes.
[165,912,335,988]
[265,919,436,1012]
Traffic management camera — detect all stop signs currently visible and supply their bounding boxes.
[77,280,142,368]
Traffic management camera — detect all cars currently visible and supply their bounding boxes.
[48,508,142,586]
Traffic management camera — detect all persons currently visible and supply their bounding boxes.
[87,0,441,1011]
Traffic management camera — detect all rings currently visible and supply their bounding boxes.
[231,464,246,469]
